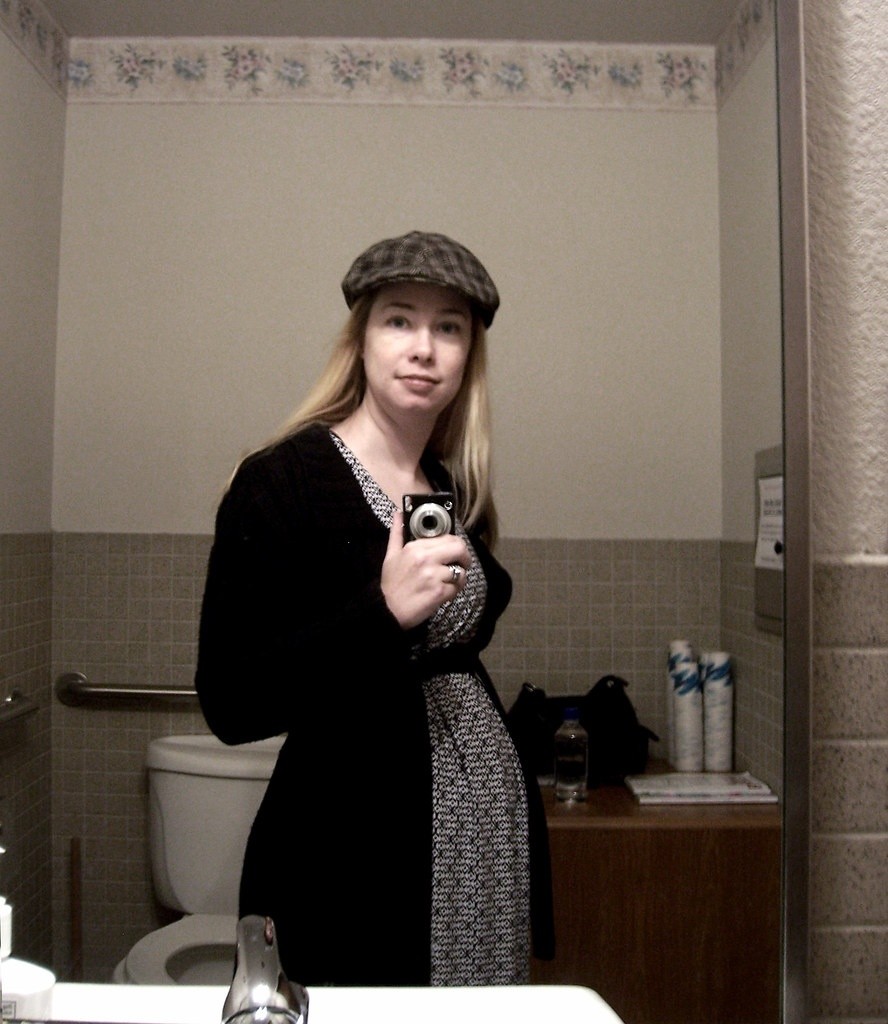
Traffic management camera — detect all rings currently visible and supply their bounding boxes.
[448,564,461,584]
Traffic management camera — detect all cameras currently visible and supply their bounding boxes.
[402,492,456,547]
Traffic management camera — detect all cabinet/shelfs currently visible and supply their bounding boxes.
[529,770,783,1024]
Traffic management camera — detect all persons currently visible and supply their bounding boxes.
[190,227,553,987]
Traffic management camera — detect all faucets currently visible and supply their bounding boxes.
[222,915,309,1024]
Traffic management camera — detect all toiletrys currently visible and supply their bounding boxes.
[0,847,55,1022]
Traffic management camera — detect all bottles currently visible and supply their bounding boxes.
[552,711,590,808]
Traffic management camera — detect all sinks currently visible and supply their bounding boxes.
[53,982,354,1024]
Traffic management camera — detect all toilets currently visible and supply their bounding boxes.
[115,736,284,985]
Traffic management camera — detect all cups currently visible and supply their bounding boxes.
[664,639,732,772]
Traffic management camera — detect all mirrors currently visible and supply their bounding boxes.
[0,0,811,1024]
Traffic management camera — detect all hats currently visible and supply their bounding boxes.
[340,230,500,329]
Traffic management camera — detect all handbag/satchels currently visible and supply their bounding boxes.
[504,675,659,780]
